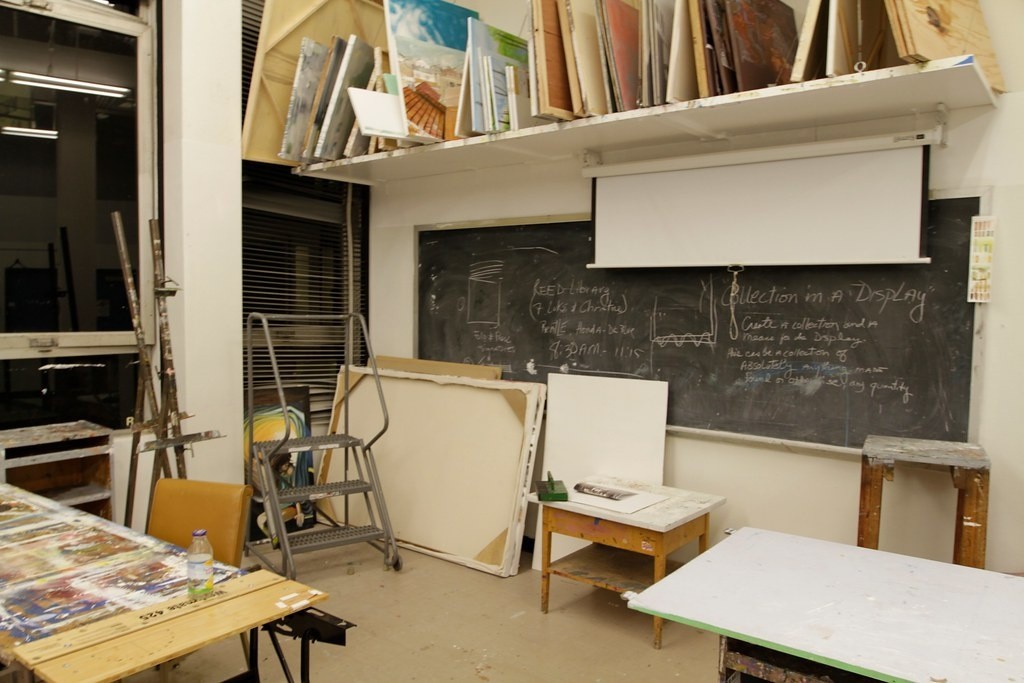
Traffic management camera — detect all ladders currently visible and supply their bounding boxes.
[239,310,401,587]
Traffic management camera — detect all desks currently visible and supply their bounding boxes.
[626,525,1024,682]
[526,472,727,649]
[1,481,358,683]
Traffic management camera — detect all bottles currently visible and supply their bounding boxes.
[186,529,213,599]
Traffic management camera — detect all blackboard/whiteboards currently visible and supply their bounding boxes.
[412,182,998,464]
